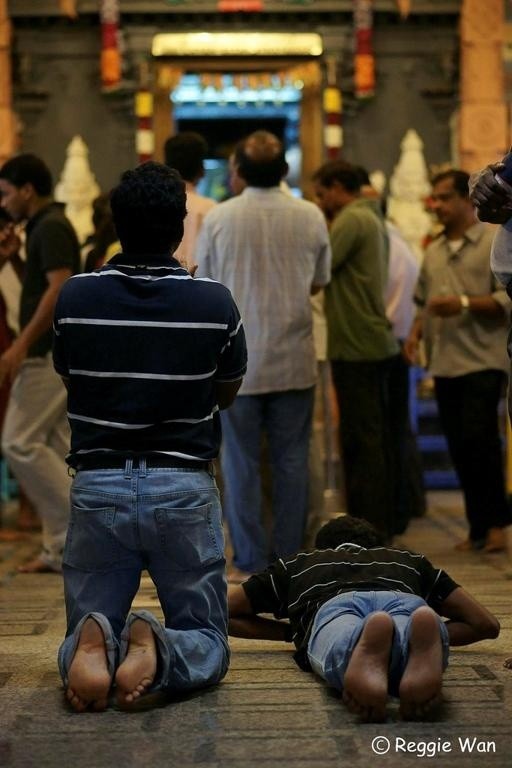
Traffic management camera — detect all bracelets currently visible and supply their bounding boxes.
[460,292,471,320]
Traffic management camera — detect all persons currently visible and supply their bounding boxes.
[1,131,426,583]
[50,161,247,713]
[469,153,512,301]
[227,516,500,724]
[402,169,512,552]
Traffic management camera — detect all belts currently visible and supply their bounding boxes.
[71,452,210,469]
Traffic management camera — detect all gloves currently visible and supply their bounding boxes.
[469,160,506,210]
